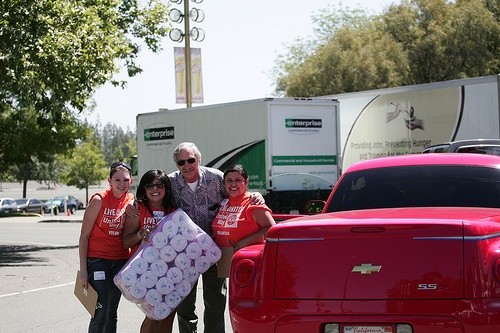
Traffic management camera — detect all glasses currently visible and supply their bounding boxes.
[111,162,132,178]
[145,182,165,191]
[176,156,198,166]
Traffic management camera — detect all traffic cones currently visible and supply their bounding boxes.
[67,209,71,217]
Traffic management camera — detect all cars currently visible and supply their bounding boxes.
[1,199,49,216]
[228,151,500,333]
[44,198,77,213]
[0,197,13,207]
[54,195,84,210]
[423,138,500,157]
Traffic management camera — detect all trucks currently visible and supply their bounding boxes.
[116,96,343,222]
[336,74,500,174]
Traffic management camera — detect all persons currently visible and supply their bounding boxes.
[122,169,177,333]
[212,165,276,253]
[79,162,135,333]
[126,143,265,333]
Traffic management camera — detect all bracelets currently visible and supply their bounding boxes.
[137,230,143,240]
